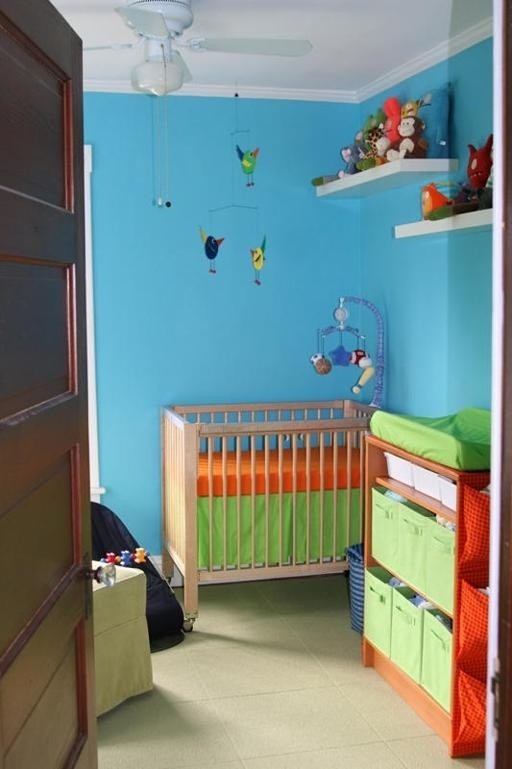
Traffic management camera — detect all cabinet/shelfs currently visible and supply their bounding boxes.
[319,158,492,240]
[360,433,492,759]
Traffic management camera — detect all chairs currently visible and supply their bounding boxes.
[93,559,155,719]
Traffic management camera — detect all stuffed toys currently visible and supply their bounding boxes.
[419,134,491,221]
[310,81,450,187]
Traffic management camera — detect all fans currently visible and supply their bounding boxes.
[83,0,313,209]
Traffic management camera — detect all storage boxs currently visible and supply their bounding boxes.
[364,487,455,718]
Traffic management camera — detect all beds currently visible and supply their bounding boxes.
[161,398,381,632]
